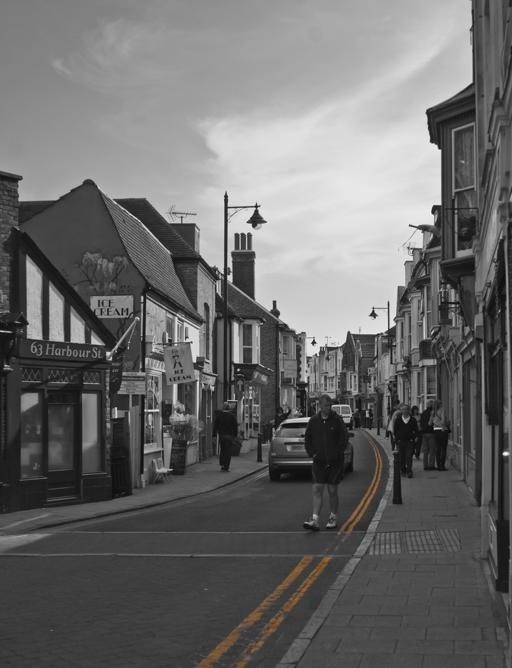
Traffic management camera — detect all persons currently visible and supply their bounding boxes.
[211,401,240,472]
[349,402,454,480]
[274,402,291,431]
[303,394,349,531]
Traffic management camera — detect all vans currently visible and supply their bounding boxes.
[330,404,353,429]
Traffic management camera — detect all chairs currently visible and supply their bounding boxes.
[152,457,174,485]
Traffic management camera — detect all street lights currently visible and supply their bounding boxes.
[221,190,267,402]
[368,301,393,366]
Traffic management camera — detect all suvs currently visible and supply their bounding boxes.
[268,416,354,482]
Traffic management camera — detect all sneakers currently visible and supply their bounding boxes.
[326,515,337,529]
[221,466,231,472]
[401,471,413,478]
[424,467,448,471]
[303,518,320,531]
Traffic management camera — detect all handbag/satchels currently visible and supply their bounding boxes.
[230,439,242,456]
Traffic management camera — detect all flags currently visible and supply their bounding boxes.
[163,343,196,386]
